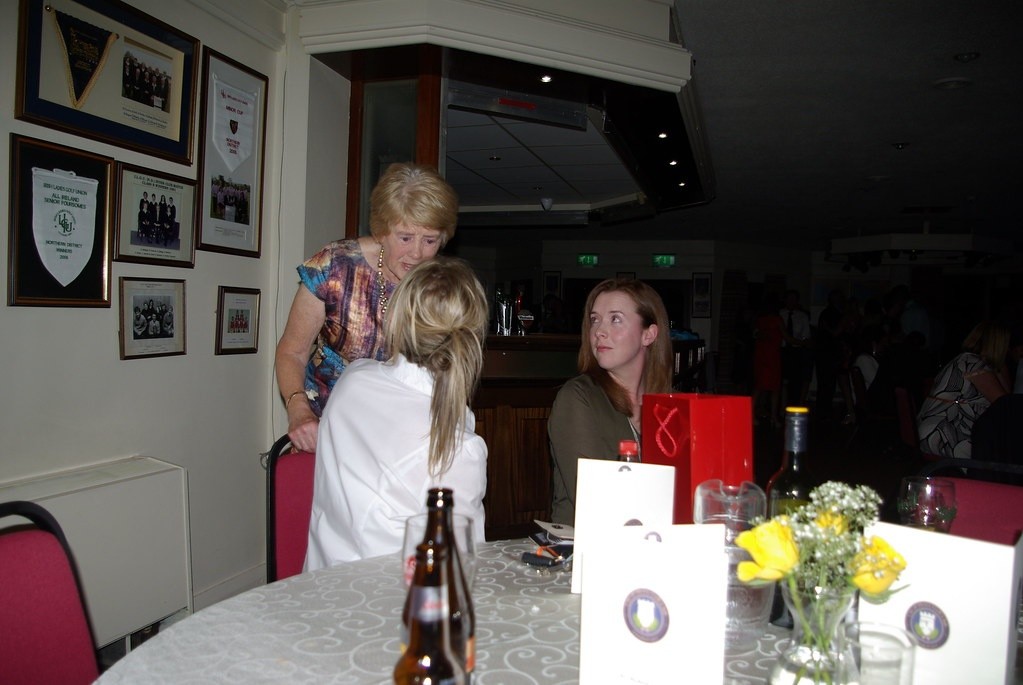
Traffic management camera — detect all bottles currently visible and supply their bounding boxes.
[617,438,641,463]
[404,486,477,684]
[766,407,814,627]
[392,546,468,685]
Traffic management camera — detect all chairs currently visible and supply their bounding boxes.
[845,371,899,449]
[0,501,105,685]
[265,433,315,584]
[913,458,1023,547]
[896,382,945,462]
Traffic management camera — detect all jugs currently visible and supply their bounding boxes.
[694,479,774,630]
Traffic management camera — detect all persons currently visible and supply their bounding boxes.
[230,310,248,332]
[729,284,1023,474]
[123,51,169,111]
[133,298,174,340]
[211,175,250,225]
[138,191,176,246]
[275,161,457,453]
[302,253,489,573]
[547,276,680,527]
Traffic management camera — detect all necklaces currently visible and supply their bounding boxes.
[378,245,388,313]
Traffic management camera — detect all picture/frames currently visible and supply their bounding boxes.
[113,160,198,268]
[195,45,269,258]
[14,0,201,164]
[215,286,261,354]
[616,272,635,279]
[543,271,561,314]
[691,272,712,319]
[118,276,188,361]
[7,133,114,308]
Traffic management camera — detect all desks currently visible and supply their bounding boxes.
[92,537,1023,685]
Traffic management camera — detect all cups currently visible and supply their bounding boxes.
[897,477,955,534]
[837,620,917,685]
[405,513,475,600]
[496,301,512,335]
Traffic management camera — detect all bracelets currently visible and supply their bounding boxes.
[285,391,307,409]
